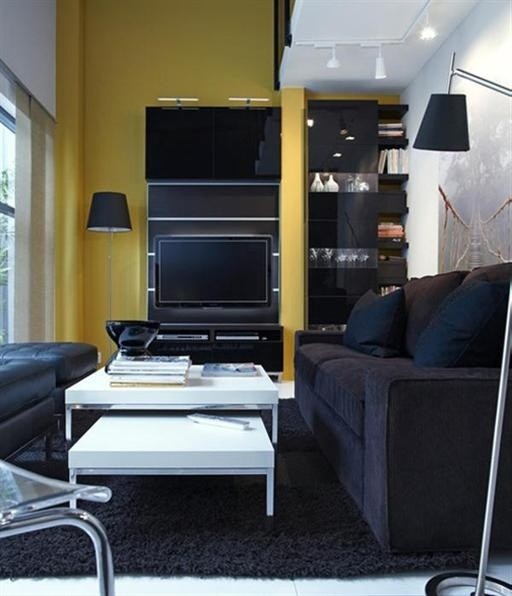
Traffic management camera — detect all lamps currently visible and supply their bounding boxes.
[420,7,438,43]
[226,95,271,106]
[85,187,136,360]
[371,42,389,82]
[156,96,199,106]
[323,39,343,70]
[411,48,512,152]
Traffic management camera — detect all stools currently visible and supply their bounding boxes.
[2,457,122,596]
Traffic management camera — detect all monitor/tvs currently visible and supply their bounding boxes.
[153,233,271,308]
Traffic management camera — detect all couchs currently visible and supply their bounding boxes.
[0,339,99,432]
[0,359,61,466]
[291,261,512,554]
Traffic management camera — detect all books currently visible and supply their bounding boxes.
[108,357,191,386]
[202,362,257,378]
[377,122,410,175]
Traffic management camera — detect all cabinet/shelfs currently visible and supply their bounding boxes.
[375,103,414,282]
[308,101,383,328]
[144,105,282,186]
[144,321,286,384]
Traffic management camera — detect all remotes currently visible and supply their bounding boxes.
[186,412,249,431]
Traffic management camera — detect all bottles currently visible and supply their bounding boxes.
[311,172,370,192]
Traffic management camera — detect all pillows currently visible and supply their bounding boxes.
[343,282,409,358]
[403,271,472,354]
[410,264,511,368]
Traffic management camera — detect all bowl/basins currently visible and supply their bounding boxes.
[105,320,160,359]
[307,323,347,332]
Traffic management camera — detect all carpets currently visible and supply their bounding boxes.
[1,394,483,583]
